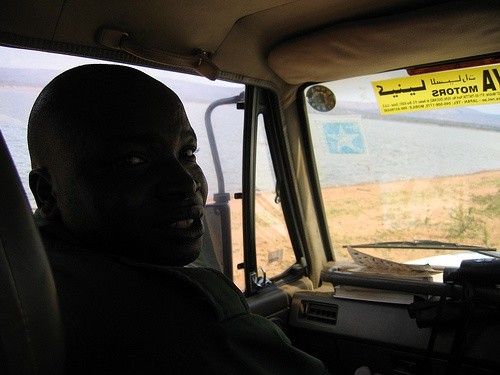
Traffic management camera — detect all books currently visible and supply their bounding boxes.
[346,244,445,284]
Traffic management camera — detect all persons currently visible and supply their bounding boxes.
[27,64,331,375]
